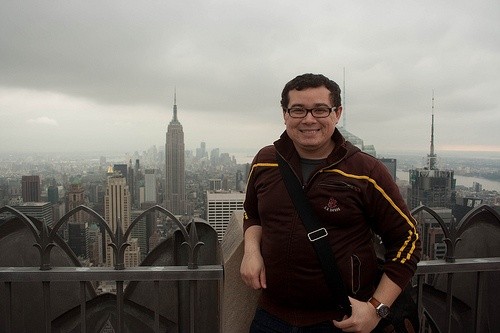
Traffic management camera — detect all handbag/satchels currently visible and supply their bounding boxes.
[347,272,419,333]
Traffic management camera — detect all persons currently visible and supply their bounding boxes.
[238,71,424,333]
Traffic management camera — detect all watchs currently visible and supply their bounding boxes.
[369,297,391,319]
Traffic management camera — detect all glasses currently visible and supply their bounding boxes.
[282,106,338,118]
